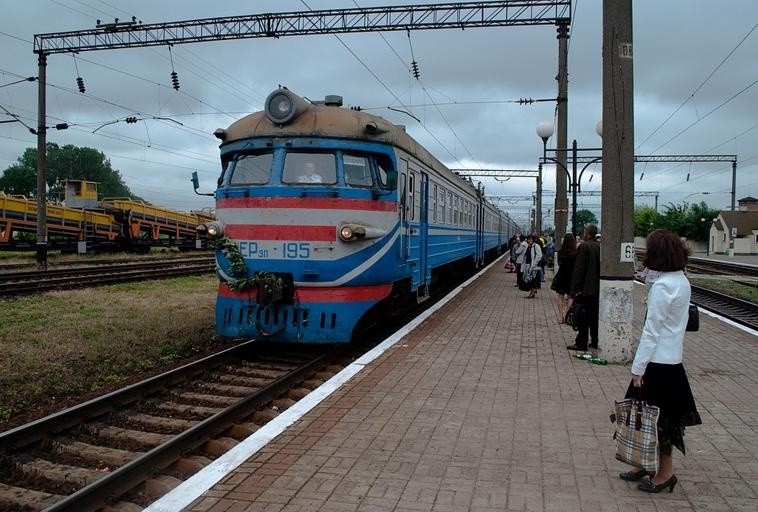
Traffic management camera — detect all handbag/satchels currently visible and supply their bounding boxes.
[685,304,700,332]
[538,260,548,268]
[564,306,586,331]
[613,379,661,477]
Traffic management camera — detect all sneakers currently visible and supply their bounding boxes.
[588,342,598,349]
[566,344,589,352]
[524,291,538,300]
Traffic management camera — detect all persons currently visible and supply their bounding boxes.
[610,228,702,493]
[508,223,600,351]
[296,161,323,183]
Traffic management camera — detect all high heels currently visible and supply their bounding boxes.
[619,471,680,493]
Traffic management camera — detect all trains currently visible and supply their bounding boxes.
[191,84,523,343]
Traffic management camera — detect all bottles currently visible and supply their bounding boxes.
[573,354,607,365]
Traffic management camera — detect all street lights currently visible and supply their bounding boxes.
[536,121,602,241]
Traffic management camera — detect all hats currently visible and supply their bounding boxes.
[583,221,598,236]
